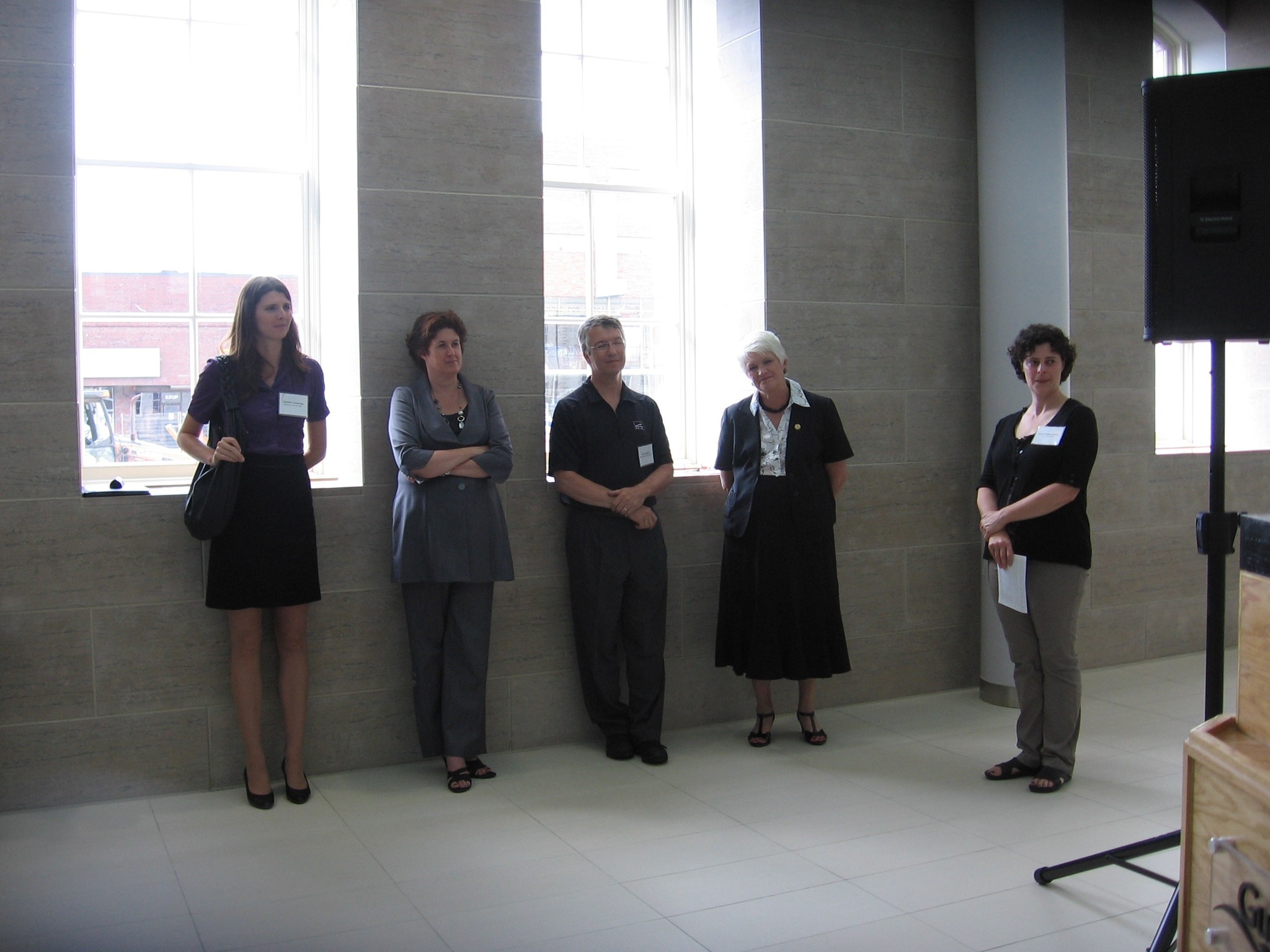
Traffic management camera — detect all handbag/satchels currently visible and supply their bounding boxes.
[183,355,244,542]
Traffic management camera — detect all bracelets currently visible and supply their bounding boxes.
[442,470,451,477]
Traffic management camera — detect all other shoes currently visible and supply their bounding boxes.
[606,734,634,760]
[634,741,668,765]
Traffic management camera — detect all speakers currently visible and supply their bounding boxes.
[1139,65,1270,346]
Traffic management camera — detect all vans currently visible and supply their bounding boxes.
[84,389,129,463]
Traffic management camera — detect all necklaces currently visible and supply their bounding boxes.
[427,379,466,430]
[758,382,792,414]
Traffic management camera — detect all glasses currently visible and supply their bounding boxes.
[590,339,625,350]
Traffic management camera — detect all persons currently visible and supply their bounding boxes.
[174,275,331,810]
[977,324,1099,795]
[713,329,854,748]
[546,314,674,765]
[388,309,518,792]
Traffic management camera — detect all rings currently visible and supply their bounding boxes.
[623,507,628,512]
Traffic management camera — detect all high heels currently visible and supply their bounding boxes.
[282,758,310,804]
[747,711,775,747]
[243,767,274,810]
[796,709,827,746]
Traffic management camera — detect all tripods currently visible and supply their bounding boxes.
[1033,336,1246,952]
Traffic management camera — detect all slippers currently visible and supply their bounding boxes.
[465,757,496,779]
[441,753,473,793]
[1028,766,1072,793]
[984,757,1042,780]
